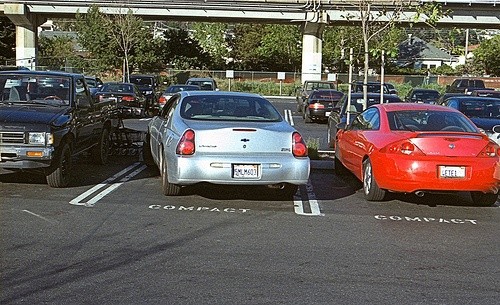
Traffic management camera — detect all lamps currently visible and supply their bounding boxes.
[327,73,337,83]
[277,71,286,94]
[226,70,235,90]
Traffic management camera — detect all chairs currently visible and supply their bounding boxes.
[8,86,28,102]
[188,107,212,115]
[234,106,256,115]
[399,125,422,130]
[44,87,75,101]
[442,125,465,131]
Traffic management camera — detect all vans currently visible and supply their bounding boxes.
[186,78,218,91]
[125,73,161,107]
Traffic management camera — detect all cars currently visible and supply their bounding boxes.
[158,84,201,115]
[144,90,311,196]
[302,89,345,124]
[95,82,148,117]
[486,124,500,148]
[435,93,470,106]
[334,102,500,207]
[327,92,405,149]
[346,80,398,96]
[434,97,500,135]
[84,76,105,102]
[404,89,440,105]
[470,90,500,100]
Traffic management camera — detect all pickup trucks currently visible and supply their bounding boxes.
[444,78,495,95]
[295,80,336,112]
[0,69,119,188]
